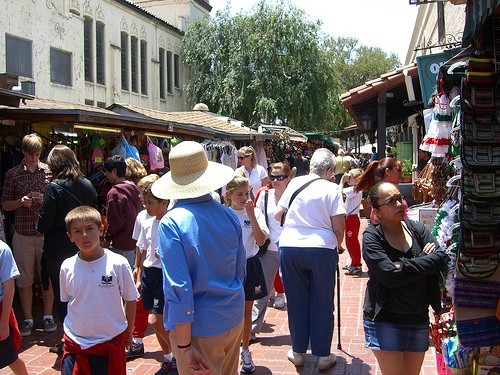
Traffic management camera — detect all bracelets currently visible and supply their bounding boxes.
[177,342,191,348]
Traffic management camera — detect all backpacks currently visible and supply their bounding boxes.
[80,132,107,168]
[146,135,181,170]
[112,135,141,163]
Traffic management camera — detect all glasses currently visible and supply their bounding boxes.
[238,155,250,160]
[376,195,403,208]
[297,153,301,155]
[269,173,288,181]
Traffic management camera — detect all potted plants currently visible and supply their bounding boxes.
[400,157,413,183]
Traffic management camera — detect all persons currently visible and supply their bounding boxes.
[361,181,452,375]
[0,236,29,375]
[354,156,410,230]
[222,146,400,212]
[220,176,269,375]
[98,153,145,270]
[237,161,297,351]
[411,145,438,182]
[0,131,186,375]
[274,147,349,372]
[150,140,249,375]
[54,204,142,375]
[336,167,368,275]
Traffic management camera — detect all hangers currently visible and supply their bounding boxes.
[201,137,234,149]
[430,79,450,106]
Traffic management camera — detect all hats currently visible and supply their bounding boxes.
[150,140,235,200]
[338,149,344,154]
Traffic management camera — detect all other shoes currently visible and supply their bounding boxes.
[317,353,337,371]
[286,349,304,367]
[345,266,362,275]
[240,331,256,344]
[342,263,352,269]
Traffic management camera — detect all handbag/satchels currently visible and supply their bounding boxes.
[255,238,271,257]
[456,315,500,345]
[455,48,500,279]
[453,277,500,309]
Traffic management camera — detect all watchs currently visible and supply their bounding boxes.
[126,337,133,342]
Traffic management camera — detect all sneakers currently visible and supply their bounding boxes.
[42,318,57,332]
[18,321,34,336]
[240,349,255,373]
[154,357,179,375]
[125,341,145,357]
[267,292,285,308]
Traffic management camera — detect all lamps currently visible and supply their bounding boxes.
[71,123,122,134]
[143,131,174,139]
